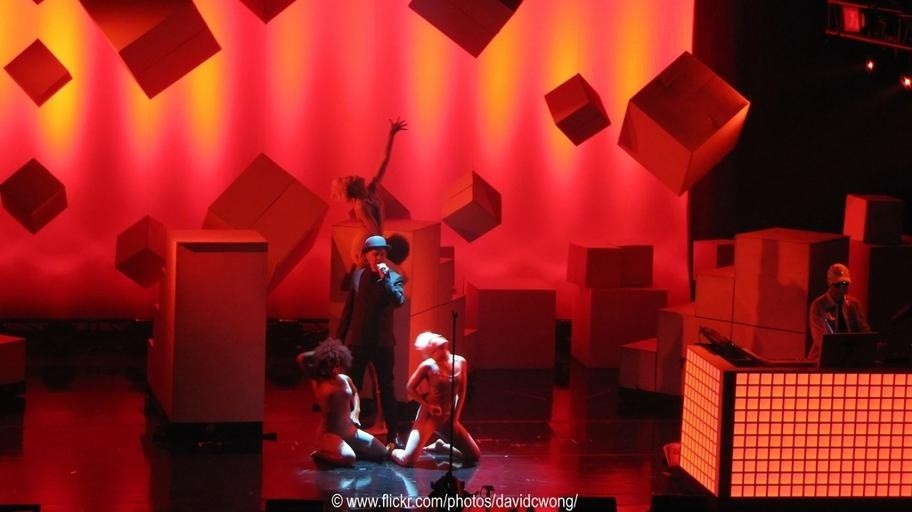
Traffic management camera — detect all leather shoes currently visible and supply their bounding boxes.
[389,436,405,449]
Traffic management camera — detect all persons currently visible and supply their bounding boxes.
[328,114,408,268]
[386,330,480,467]
[295,336,389,465]
[341,233,407,445]
[805,261,872,361]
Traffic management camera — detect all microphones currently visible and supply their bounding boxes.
[377,263,390,278]
[451,308,458,320]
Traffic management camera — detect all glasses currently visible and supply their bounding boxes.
[834,282,848,287]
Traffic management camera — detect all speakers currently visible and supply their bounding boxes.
[266,499,325,512]
[559,497,616,511]
[0,505,40,511]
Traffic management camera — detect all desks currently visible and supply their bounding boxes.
[678,340,912,511]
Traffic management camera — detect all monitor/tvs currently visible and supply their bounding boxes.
[820,333,879,373]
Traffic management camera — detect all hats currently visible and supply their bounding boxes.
[828,264,850,285]
[361,235,391,253]
